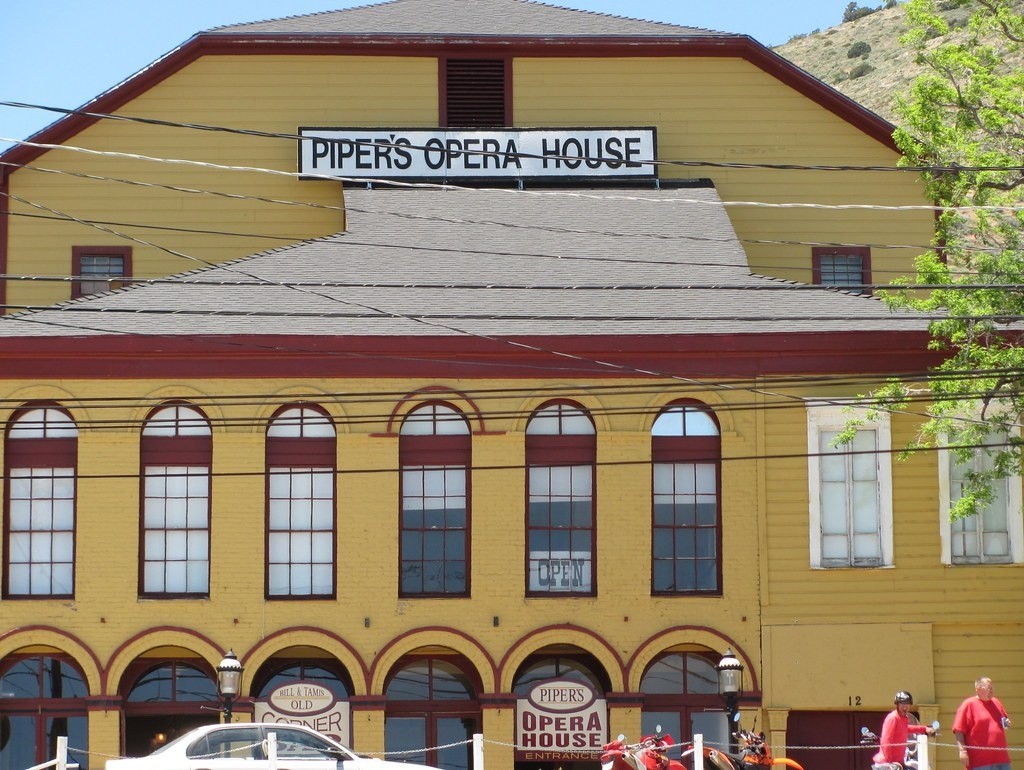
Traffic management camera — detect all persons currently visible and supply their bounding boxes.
[872,690,938,770]
[952,675,1014,770]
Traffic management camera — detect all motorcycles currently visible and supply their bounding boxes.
[683,718,805,770]
[861,713,943,770]
[600,728,683,770]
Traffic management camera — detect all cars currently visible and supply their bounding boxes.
[103,724,434,770]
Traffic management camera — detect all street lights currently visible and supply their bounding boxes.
[715,645,745,769]
[215,644,245,764]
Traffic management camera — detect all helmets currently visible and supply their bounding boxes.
[894,691,913,705]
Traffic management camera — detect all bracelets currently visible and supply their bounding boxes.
[959,750,967,753]
